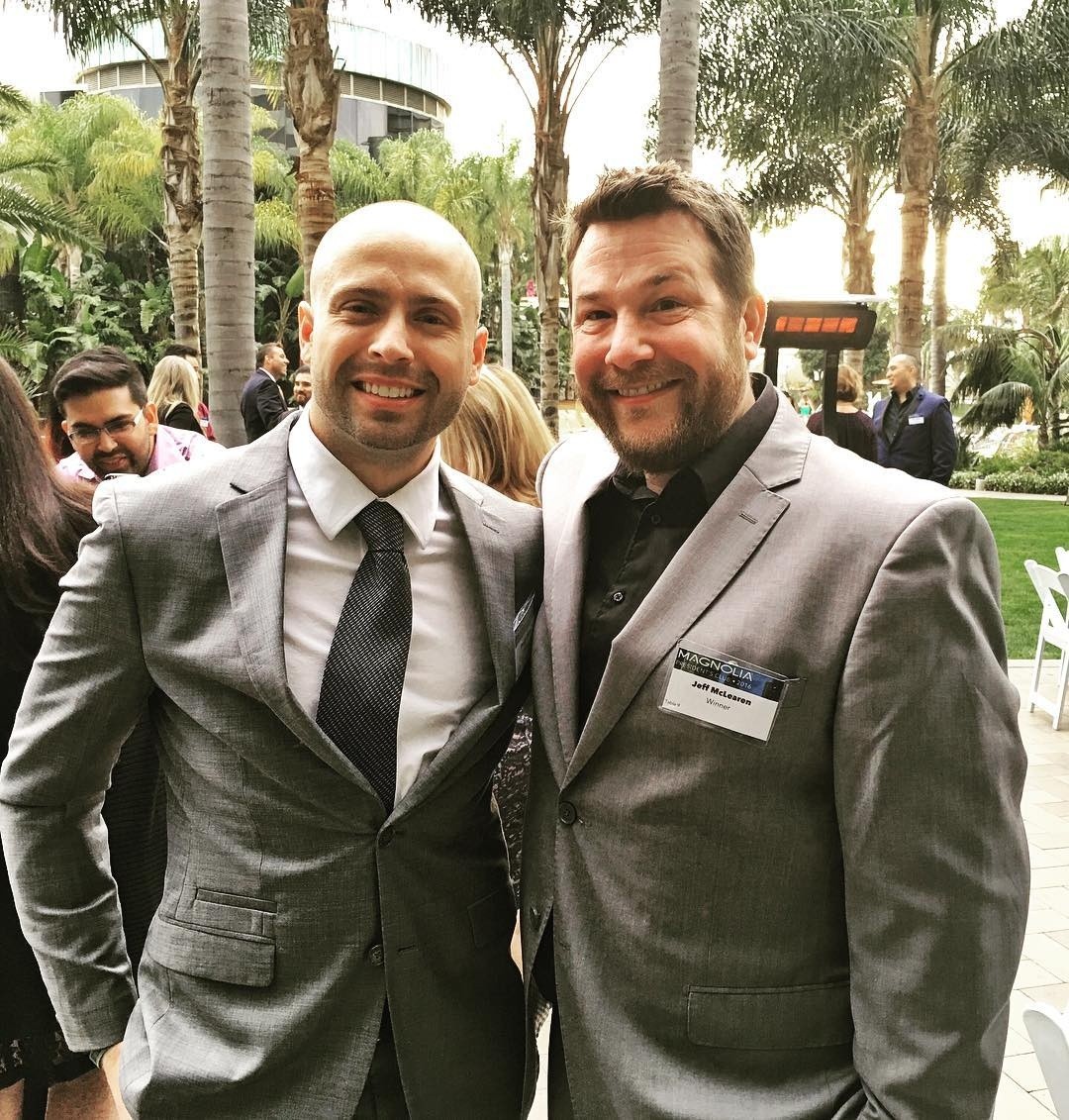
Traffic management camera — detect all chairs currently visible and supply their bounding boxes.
[1023,546,1069,731]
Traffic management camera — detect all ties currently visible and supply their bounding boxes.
[316,502,413,819]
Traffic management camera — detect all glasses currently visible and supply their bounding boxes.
[68,404,145,445]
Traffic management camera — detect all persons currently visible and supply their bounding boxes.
[806,355,958,486]
[52,346,227,489]
[520,164,1031,1120]
[437,363,556,984]
[1,356,165,1119]
[146,345,219,442]
[240,344,312,444]
[1,200,543,1119]
